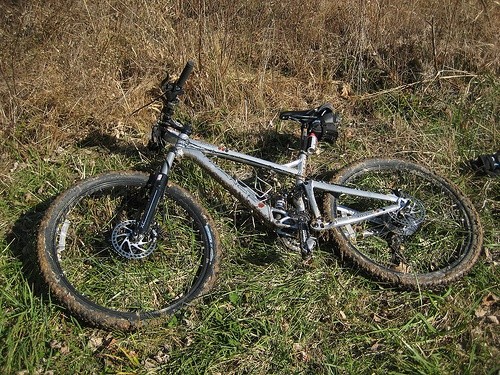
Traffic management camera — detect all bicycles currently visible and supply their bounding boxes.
[31,61,483,333]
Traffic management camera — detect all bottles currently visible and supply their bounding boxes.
[275,193,285,209]
